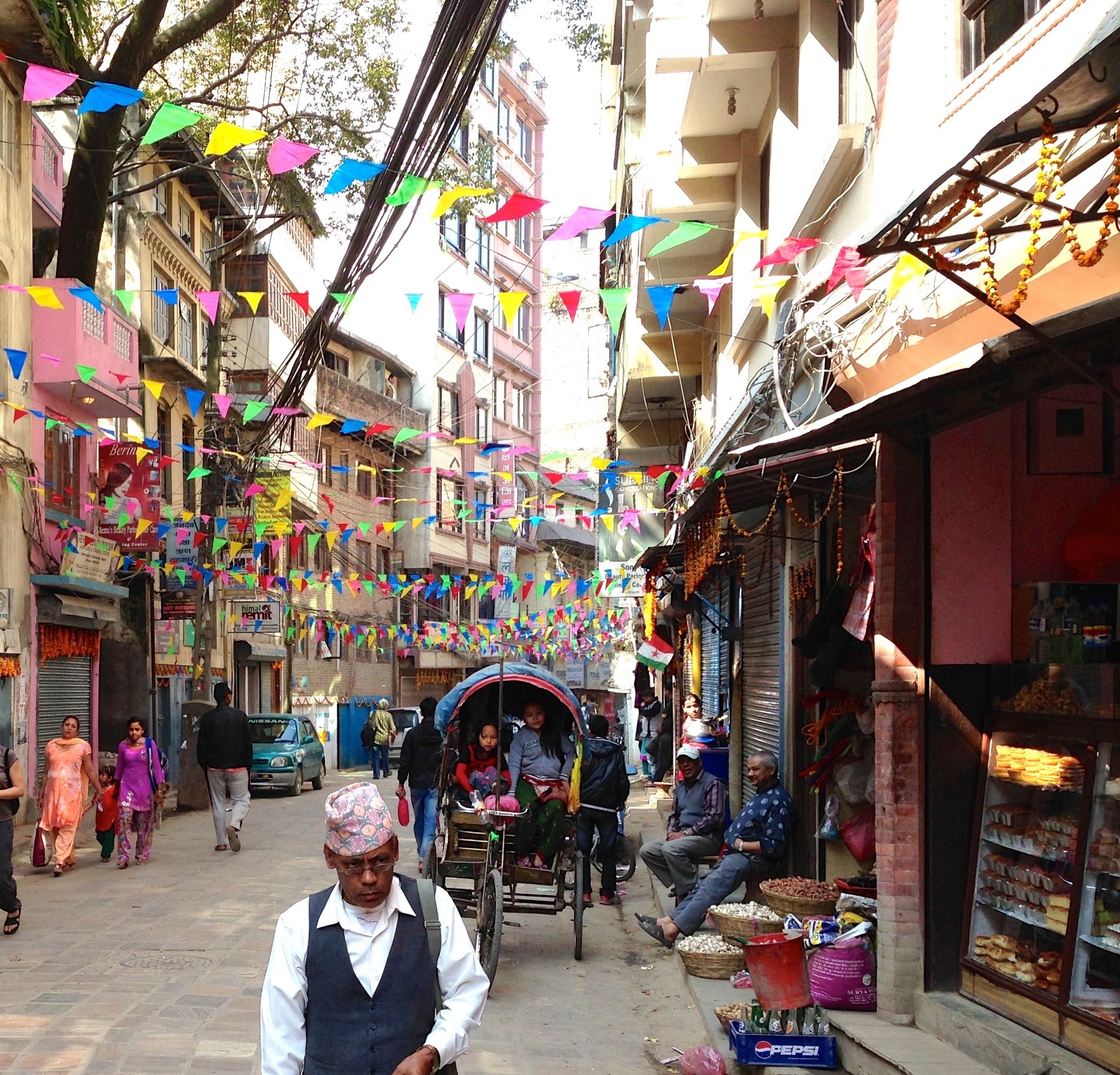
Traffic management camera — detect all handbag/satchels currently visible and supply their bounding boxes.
[29,810,53,868]
[4,749,20,815]
[146,737,170,792]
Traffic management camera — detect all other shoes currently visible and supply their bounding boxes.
[53,864,63,877]
[214,844,227,851]
[599,895,621,905]
[225,826,241,852]
[137,859,144,865]
[102,857,110,862]
[116,860,128,869]
[667,886,677,897]
[373,776,379,779]
[570,890,591,903]
[61,863,76,870]
[383,771,392,777]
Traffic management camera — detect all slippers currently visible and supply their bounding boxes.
[637,919,674,950]
[535,860,548,869]
[516,856,531,866]
[635,911,658,928]
[3,905,22,935]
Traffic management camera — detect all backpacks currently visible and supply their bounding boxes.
[360,710,377,754]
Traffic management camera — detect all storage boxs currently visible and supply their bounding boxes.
[727,1018,838,1071]
[832,876,877,898]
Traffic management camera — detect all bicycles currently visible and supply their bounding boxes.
[553,814,636,888]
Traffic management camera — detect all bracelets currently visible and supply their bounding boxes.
[37,797,44,801]
[158,790,163,792]
[415,1045,439,1075]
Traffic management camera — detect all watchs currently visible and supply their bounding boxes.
[738,841,744,851]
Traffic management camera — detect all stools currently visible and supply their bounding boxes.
[675,855,720,909]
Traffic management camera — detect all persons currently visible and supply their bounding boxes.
[395,696,444,873]
[635,749,796,949]
[639,746,726,899]
[452,720,511,803]
[197,683,253,852]
[365,698,396,779]
[84,765,120,863]
[581,682,729,788]
[505,698,579,872]
[576,715,630,905]
[114,716,165,870]
[97,461,142,525]
[0,743,25,935]
[37,716,104,877]
[259,782,491,1075]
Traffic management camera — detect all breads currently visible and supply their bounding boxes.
[972,933,1068,998]
[979,854,1120,938]
[994,745,1085,790]
[984,801,1120,873]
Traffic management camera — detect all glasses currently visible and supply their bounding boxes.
[227,690,233,696]
[336,850,397,877]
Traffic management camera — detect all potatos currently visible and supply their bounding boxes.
[715,1002,752,1019]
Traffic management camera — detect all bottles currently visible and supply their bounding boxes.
[738,999,831,1035]
[1027,593,1115,664]
[469,789,494,832]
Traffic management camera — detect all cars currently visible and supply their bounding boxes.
[248,713,326,796]
[369,707,421,769]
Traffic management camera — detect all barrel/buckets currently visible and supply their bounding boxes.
[740,932,811,1010]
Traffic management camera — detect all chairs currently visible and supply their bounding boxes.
[257,728,297,742]
[398,716,408,727]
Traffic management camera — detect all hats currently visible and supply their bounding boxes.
[325,781,395,856]
[676,746,700,759]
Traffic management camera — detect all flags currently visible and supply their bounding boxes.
[635,632,675,671]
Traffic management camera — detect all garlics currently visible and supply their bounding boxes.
[676,934,744,954]
[710,900,784,921]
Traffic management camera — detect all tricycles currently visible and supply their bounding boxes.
[428,662,593,994]
